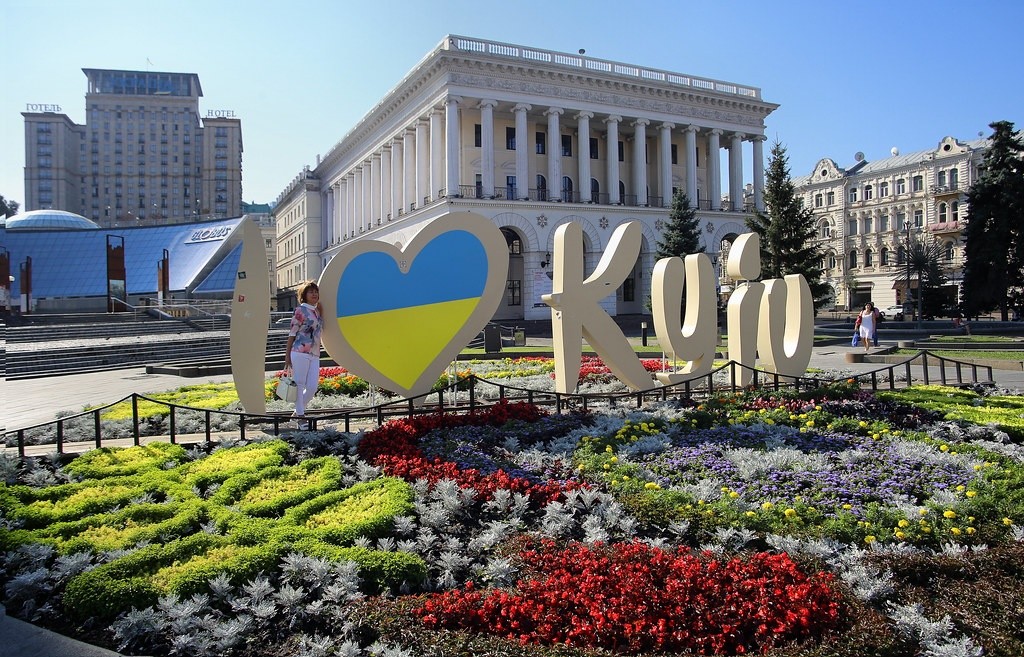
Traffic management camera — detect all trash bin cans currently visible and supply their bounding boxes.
[483,324,502,354]
[513,327,526,347]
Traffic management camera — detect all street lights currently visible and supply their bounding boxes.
[900,221,923,325]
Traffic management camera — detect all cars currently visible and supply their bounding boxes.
[879,304,903,315]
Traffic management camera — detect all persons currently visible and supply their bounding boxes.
[954,315,970,333]
[286,280,323,429]
[854,302,879,351]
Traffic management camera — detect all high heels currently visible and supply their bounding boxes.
[289,413,312,430]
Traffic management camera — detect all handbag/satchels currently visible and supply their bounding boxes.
[855,309,863,324]
[276,363,298,401]
[851,331,859,348]
